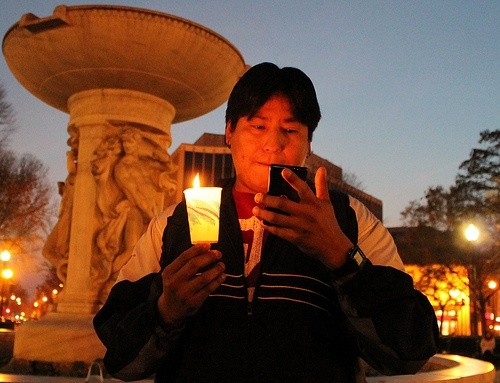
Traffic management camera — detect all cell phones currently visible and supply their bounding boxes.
[262,163,308,227]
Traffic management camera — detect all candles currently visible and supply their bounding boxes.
[183,173,223,244]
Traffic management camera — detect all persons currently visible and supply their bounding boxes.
[110,128,178,277]
[42,150,76,276]
[93,61,441,383]
[480,331,496,363]
